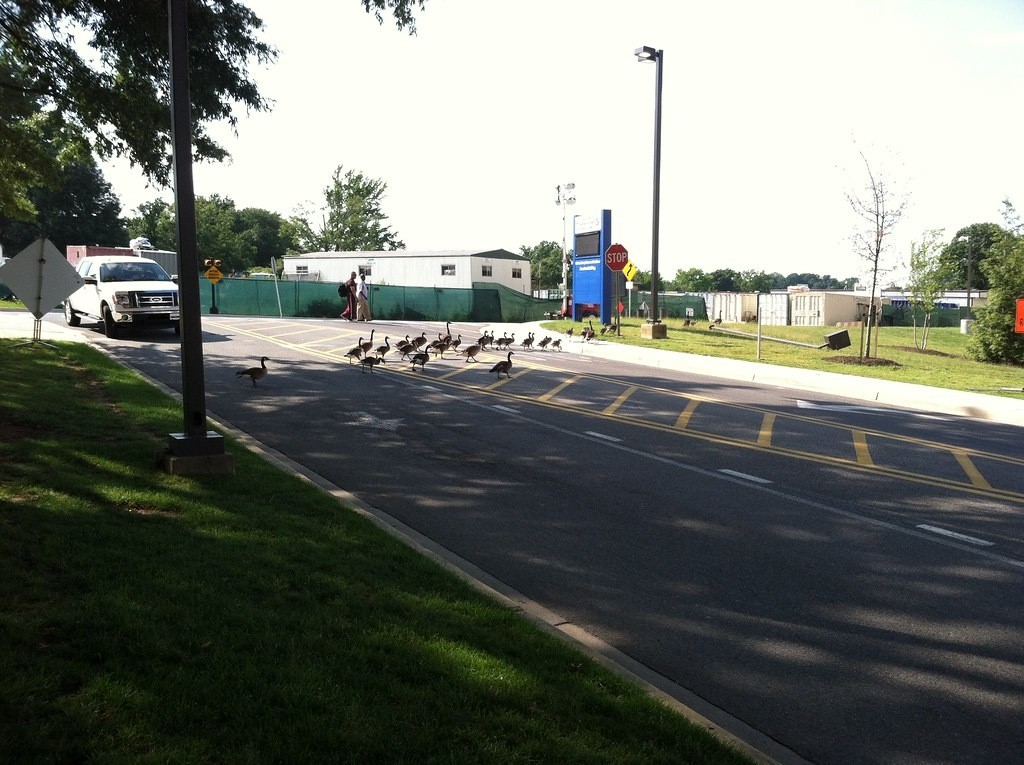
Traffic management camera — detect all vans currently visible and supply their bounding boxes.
[561,295,616,319]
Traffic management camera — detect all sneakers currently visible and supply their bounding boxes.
[357,319,365,322]
[347,317,355,323]
[366,318,374,323]
[340,314,349,320]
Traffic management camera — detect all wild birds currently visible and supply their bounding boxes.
[344,321,562,374]
[489,352,515,379]
[682,310,723,327]
[564,320,618,344]
[235,356,271,383]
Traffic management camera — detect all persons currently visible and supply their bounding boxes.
[340,272,358,321]
[355,273,374,322]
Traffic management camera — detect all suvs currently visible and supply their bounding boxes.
[62,254,179,340]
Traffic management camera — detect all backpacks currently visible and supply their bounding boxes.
[338,283,348,297]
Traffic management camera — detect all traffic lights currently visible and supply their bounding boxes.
[205,260,212,267]
[214,260,222,266]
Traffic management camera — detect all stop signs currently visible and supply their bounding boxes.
[604,244,628,271]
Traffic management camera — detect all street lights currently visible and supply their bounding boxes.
[633,46,665,322]
[709,325,853,351]
